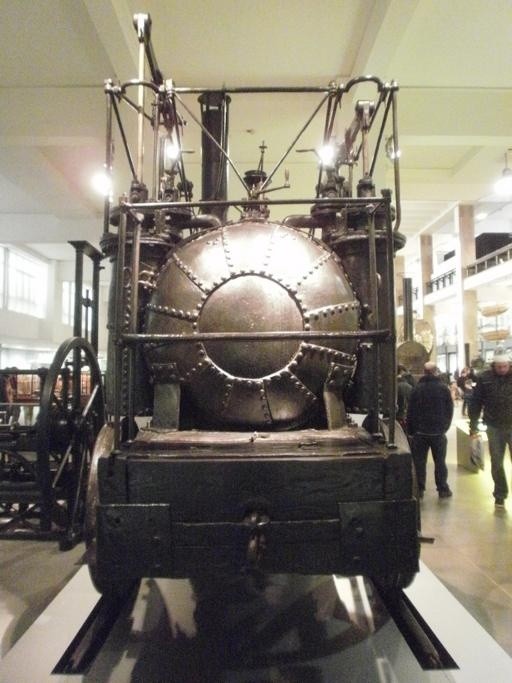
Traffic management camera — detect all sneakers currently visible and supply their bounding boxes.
[495,495,504,505]
[419,488,423,497]
[439,489,452,498]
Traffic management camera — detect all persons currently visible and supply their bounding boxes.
[406,361,454,497]
[467,354,511,507]
[397,355,492,442]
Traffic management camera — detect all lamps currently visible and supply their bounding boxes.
[494,152,512,200]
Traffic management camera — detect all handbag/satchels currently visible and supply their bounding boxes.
[470,438,484,471]
